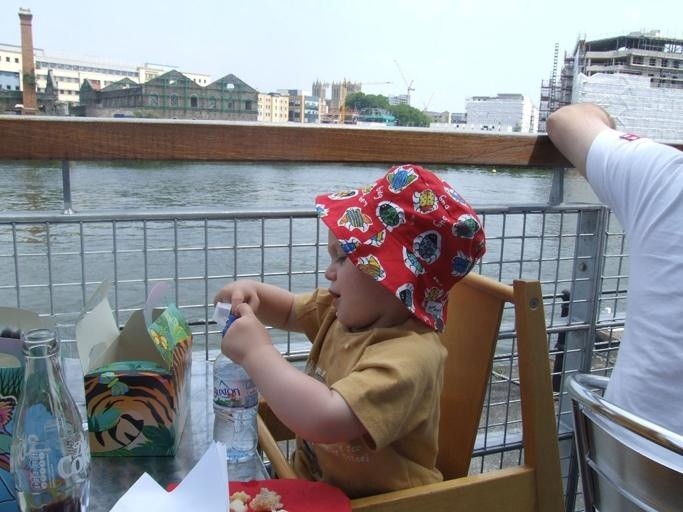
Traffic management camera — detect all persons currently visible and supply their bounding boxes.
[547,103,683,469]
[213,165,484,497]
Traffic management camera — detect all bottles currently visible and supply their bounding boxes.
[10,326,88,512]
[212,316,258,461]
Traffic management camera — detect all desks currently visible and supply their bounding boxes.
[57,356,290,512]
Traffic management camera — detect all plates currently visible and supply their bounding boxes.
[168,479,352,512]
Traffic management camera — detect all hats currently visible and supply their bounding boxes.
[315,163,485,333]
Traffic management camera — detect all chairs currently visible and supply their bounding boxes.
[227,277,565,512]
[566,369,679,510]
